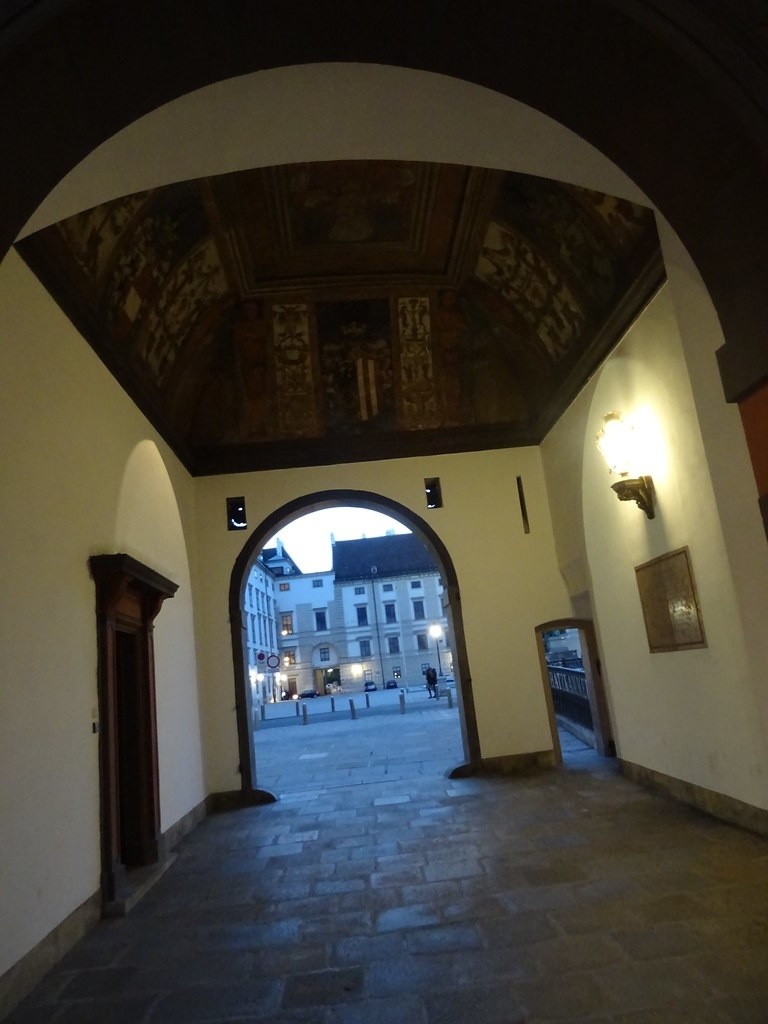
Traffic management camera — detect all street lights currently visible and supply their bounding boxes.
[429,625,443,677]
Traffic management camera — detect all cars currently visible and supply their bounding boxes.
[364,681,377,691]
[300,690,318,698]
[387,679,398,688]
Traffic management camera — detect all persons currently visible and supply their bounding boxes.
[425,667,436,699]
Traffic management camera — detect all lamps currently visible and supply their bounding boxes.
[595,412,655,521]
